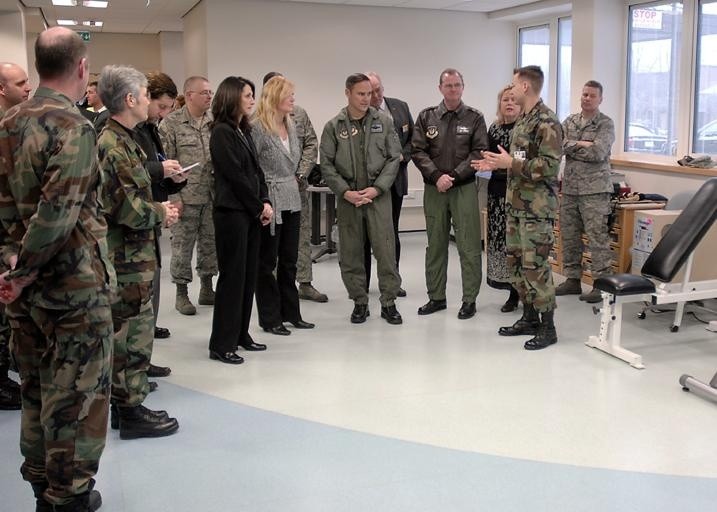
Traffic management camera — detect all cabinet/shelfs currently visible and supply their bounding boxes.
[548,194,666,290]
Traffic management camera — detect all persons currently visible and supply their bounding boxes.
[363,72,413,297]
[208,76,274,364]
[0,61,33,412]
[132,71,192,339]
[319,72,403,325]
[91,64,178,440]
[80,82,110,132]
[249,76,315,335]
[410,69,488,318]
[0,26,119,511]
[470,65,564,350]
[486,86,522,313]
[158,76,218,315]
[262,71,328,303]
[554,80,615,305]
[174,95,184,109]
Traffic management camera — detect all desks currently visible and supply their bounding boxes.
[307,184,338,264]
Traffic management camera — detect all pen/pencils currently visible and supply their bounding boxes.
[158,153,166,160]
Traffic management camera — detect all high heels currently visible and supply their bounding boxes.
[281,320,315,329]
[501,297,520,312]
[264,324,291,335]
[209,349,244,364]
[233,338,267,351]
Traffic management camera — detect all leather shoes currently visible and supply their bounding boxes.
[498,316,541,336]
[154,327,171,338]
[31,478,102,512]
[525,321,558,349]
[148,380,158,392]
[0,385,22,409]
[3,378,21,392]
[397,287,407,296]
[146,364,172,377]
[117,405,179,440]
[349,288,369,299]
[458,301,477,319]
[351,304,370,323]
[418,299,447,315]
[110,404,168,430]
[381,304,402,324]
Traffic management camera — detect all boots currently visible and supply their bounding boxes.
[198,274,216,305]
[175,283,196,315]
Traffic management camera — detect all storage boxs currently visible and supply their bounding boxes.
[631,208,716,283]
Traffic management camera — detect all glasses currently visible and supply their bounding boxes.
[186,90,214,96]
[133,92,151,100]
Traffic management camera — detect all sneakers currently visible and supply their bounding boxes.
[579,289,603,302]
[298,282,329,302]
[555,278,582,295]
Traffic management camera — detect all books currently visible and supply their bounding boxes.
[163,160,201,180]
